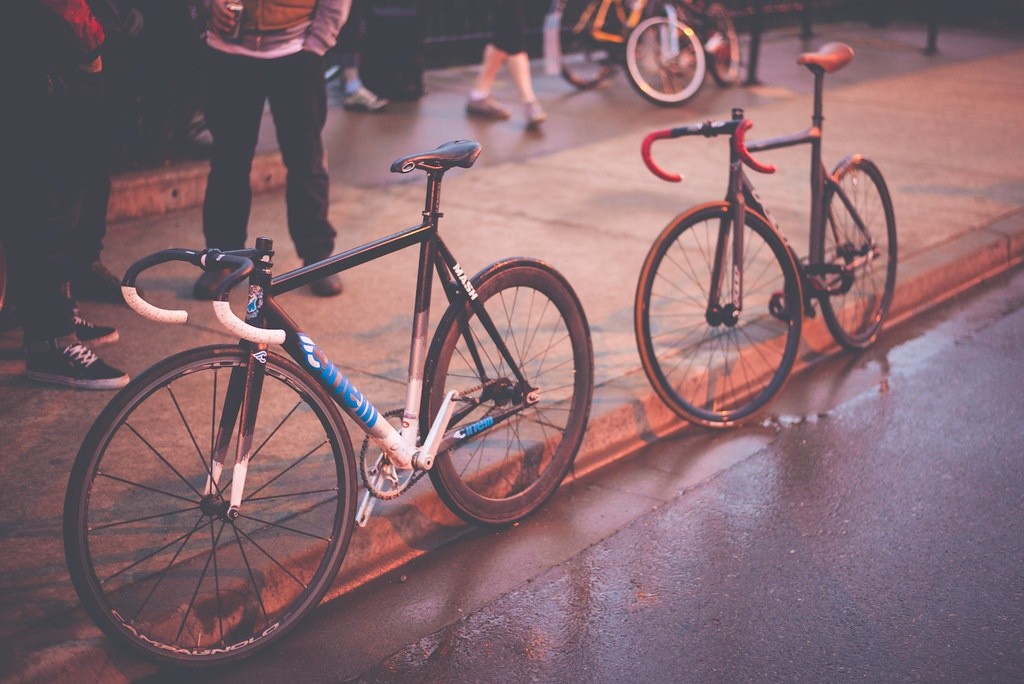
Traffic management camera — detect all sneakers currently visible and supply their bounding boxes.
[25,340,129,389]
[74,317,119,346]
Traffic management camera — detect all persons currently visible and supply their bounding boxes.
[0,0,131,389]
[188,0,353,300]
[331,1,389,112]
[467,1,547,125]
[47,0,146,306]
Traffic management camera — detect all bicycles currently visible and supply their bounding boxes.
[62,139,595,673]
[558,0,740,106]
[634,42,897,430]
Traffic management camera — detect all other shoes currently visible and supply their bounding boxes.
[343,85,389,111]
[192,267,236,299]
[465,94,513,119]
[71,263,143,306]
[524,97,548,126]
[308,273,343,296]
